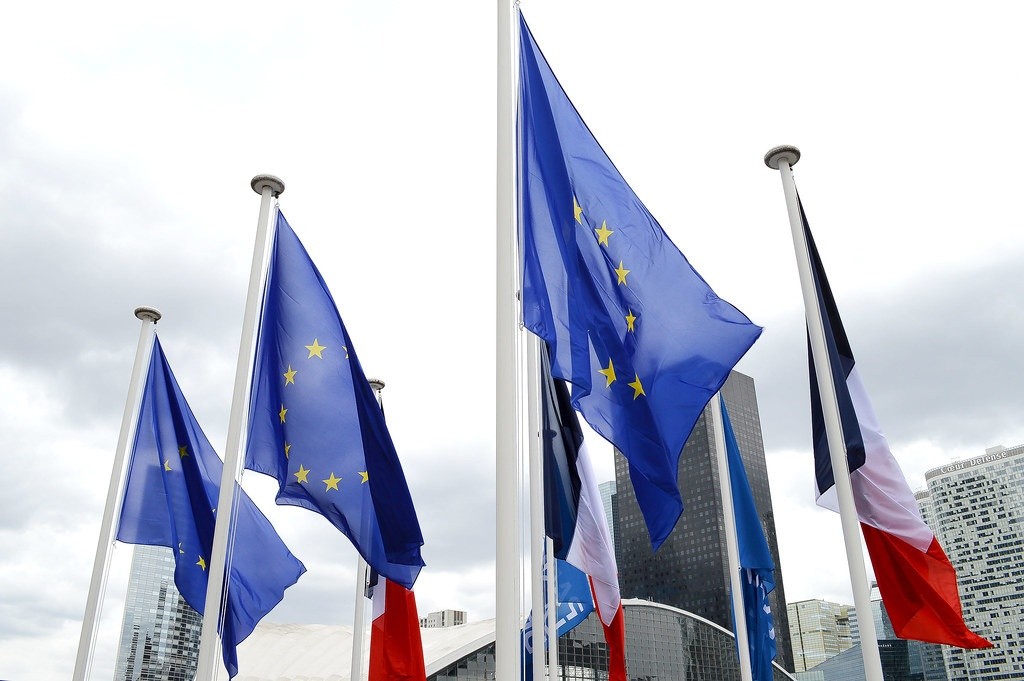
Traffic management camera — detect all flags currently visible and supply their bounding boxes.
[517,7,765,552]
[521,535,594,681]
[243,207,428,590]
[368,573,427,681]
[540,333,627,681]
[114,333,308,681]
[720,391,777,681]
[794,183,995,649]
